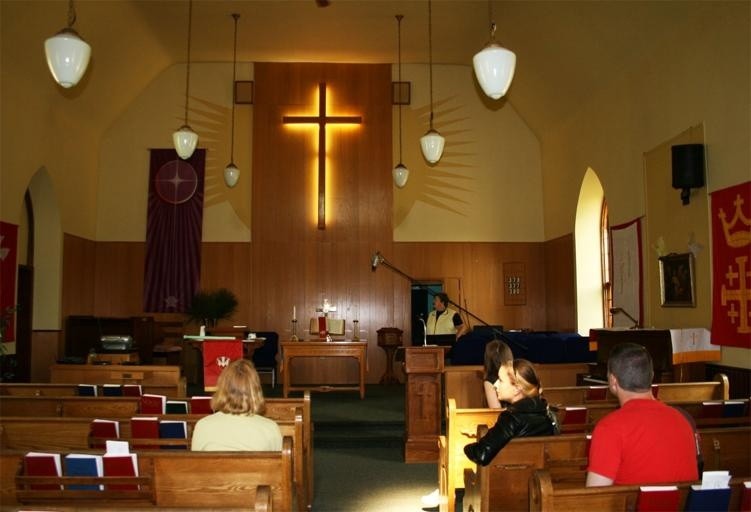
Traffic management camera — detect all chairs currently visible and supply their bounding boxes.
[247,331,278,389]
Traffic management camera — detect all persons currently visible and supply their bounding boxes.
[585,344,700,487]
[420,340,514,509]
[464,359,561,466]
[191,358,283,451]
[425,290,464,345]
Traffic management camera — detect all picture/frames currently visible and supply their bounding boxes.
[657,252,695,308]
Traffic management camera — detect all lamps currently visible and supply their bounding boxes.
[472,0,516,102]
[44,0,92,89]
[224,13,242,187]
[420,0,446,163]
[171,0,199,161]
[391,15,409,188]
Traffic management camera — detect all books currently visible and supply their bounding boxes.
[190,395,213,414]
[685,483,731,512]
[90,419,119,449]
[64,453,104,490]
[77,384,97,397]
[700,401,723,429]
[634,485,683,512]
[651,385,659,399]
[586,386,609,400]
[123,384,141,397]
[560,406,588,425]
[166,400,188,415]
[141,394,166,414]
[102,453,140,490]
[130,417,158,450]
[737,480,751,512]
[309,317,345,336]
[159,421,187,449]
[102,384,121,397]
[24,452,64,490]
[723,401,747,428]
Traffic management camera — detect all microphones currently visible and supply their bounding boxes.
[370,251,381,270]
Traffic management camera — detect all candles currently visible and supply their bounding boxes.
[354,305,357,319]
[293,305,296,319]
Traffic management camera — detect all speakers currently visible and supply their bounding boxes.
[671,143,705,190]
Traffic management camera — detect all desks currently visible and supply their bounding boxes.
[185,337,264,397]
[279,341,367,400]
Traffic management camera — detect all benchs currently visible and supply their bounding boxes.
[436,395,750,512]
[0,377,188,397]
[0,405,308,512]
[0,388,313,502]
[542,372,730,406]
[528,469,751,512]
[2,484,274,512]
[460,417,751,511]
[0,435,299,511]
